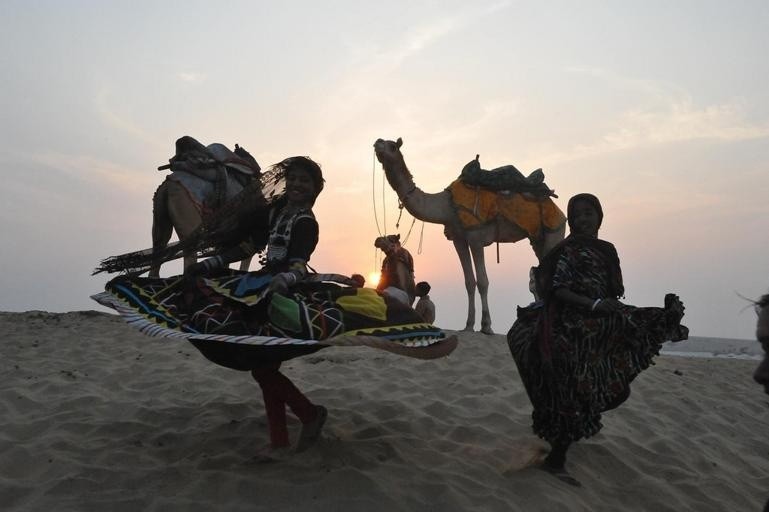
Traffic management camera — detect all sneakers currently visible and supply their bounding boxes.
[545,453,581,488]
[296,405,328,454]
[248,444,296,463]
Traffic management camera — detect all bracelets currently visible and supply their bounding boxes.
[589,297,601,311]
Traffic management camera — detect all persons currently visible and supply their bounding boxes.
[347,272,367,290]
[507,193,690,489]
[412,280,436,328]
[90,156,458,459]
